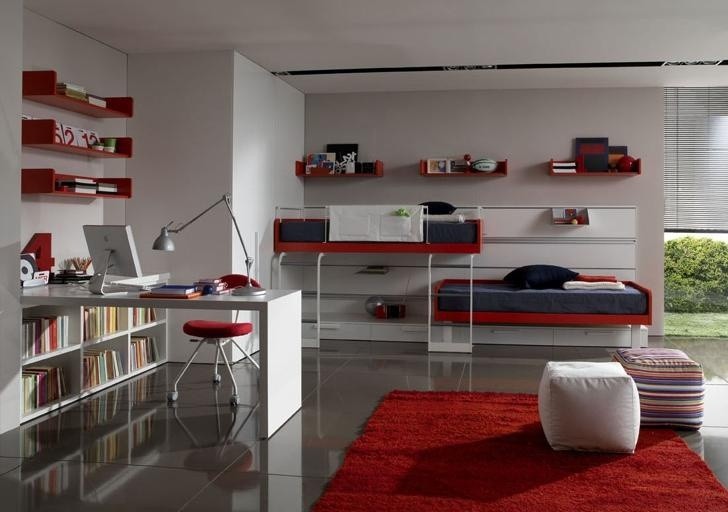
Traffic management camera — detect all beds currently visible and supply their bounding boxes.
[434,278,653,327]
[272,205,483,254]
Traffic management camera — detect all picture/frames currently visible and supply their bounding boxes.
[427,158,451,173]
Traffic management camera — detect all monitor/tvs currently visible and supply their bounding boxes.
[83,224,142,296]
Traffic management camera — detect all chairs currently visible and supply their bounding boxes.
[166,273,262,407]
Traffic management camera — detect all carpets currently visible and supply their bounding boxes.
[309,389,727,512]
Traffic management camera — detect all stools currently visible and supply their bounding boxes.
[536,345,708,455]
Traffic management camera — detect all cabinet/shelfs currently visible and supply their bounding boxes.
[22,70,135,199]
[299,323,648,349]
[18,307,169,426]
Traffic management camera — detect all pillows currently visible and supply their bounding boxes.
[501,265,579,289]
[418,201,457,215]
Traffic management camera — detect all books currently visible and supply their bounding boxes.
[553,169,577,175]
[20,305,160,419]
[194,277,232,296]
[552,161,576,167]
[21,80,119,200]
[21,368,164,508]
[140,289,202,299]
[151,283,198,296]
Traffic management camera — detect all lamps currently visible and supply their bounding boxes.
[153,193,267,296]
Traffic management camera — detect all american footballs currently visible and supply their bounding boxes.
[473,159,497,172]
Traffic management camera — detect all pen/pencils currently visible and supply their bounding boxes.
[65,259,73,266]
[71,257,94,270]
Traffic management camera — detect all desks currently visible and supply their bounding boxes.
[19,273,301,440]
